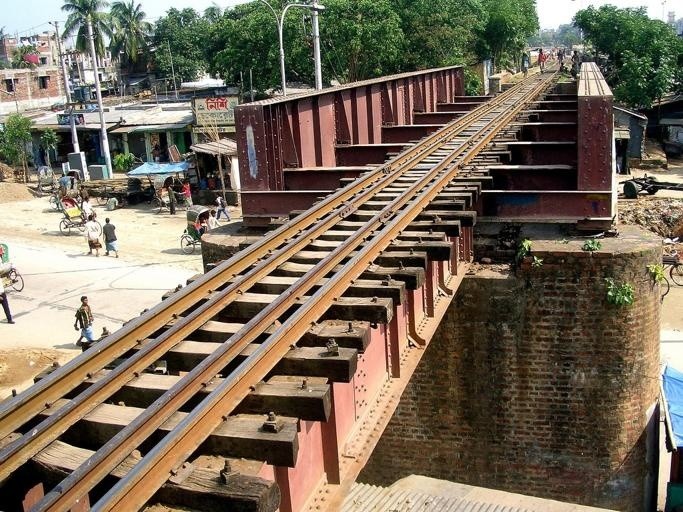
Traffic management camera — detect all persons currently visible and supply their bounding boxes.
[101,326,109,335]
[169,167,231,233]
[537,46,582,79]
[521,51,531,78]
[106,194,124,211]
[84,216,102,256]
[103,217,119,258]
[0,279,15,324]
[74,295,93,348]
[14,168,21,178]
[76,116,84,125]
[50,172,97,221]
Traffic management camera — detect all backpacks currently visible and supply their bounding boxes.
[221,199,227,207]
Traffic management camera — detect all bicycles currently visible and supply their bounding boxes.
[49,186,65,212]
[96,184,125,208]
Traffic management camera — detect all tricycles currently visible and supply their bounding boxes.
[150,175,187,215]
[59,196,102,237]
[180,205,211,254]
[0,242,24,292]
[661,236,683,296]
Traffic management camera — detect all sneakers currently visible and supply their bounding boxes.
[89,251,118,258]
[8,320,14,323]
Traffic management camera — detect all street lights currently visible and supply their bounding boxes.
[260,0,326,97]
